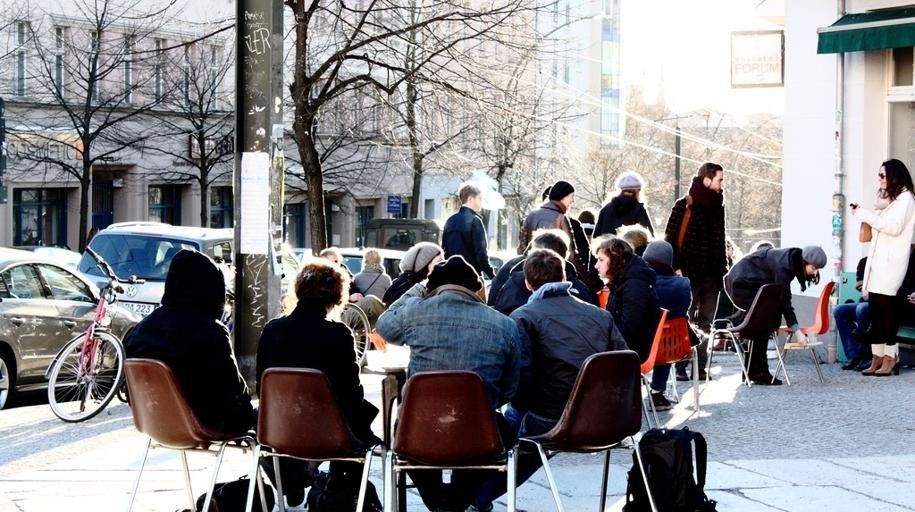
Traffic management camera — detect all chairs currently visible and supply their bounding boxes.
[705,282,792,386]
[771,281,833,384]
[245,367,388,511]
[121,358,268,511]
[384,371,520,512]
[598,278,741,429]
[514,349,659,512]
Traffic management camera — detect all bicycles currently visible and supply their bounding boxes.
[41,245,151,424]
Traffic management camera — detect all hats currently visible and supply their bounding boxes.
[548,181,575,202]
[802,246,827,268]
[398,241,444,273]
[641,240,674,266]
[614,169,642,190]
[426,254,484,294]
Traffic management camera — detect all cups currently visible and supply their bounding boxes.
[807,332,818,344]
[366,342,411,372]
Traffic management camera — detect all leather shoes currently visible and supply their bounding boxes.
[841,360,872,372]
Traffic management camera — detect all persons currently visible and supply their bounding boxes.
[639,239,699,410]
[849,159,915,377]
[721,246,828,385]
[375,256,519,512]
[321,246,394,360]
[441,184,496,285]
[382,242,445,308]
[665,164,729,381]
[588,170,654,270]
[255,262,381,512]
[621,228,712,381]
[592,238,660,363]
[121,247,305,508]
[517,181,602,299]
[86,227,100,246]
[466,249,630,512]
[493,231,593,313]
[833,243,915,372]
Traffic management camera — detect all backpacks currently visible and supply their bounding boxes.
[621,425,718,511]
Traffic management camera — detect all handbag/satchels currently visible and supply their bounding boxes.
[304,471,384,511]
[180,473,275,512]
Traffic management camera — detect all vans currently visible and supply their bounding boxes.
[72,222,302,321]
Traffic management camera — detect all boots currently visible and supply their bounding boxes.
[741,352,783,386]
[876,342,901,376]
[862,342,884,376]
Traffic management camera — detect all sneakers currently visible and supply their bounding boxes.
[674,372,713,382]
[647,389,673,412]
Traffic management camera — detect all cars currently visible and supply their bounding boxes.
[291,216,505,368]
[0,247,145,410]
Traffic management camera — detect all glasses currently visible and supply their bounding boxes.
[878,173,886,179]
